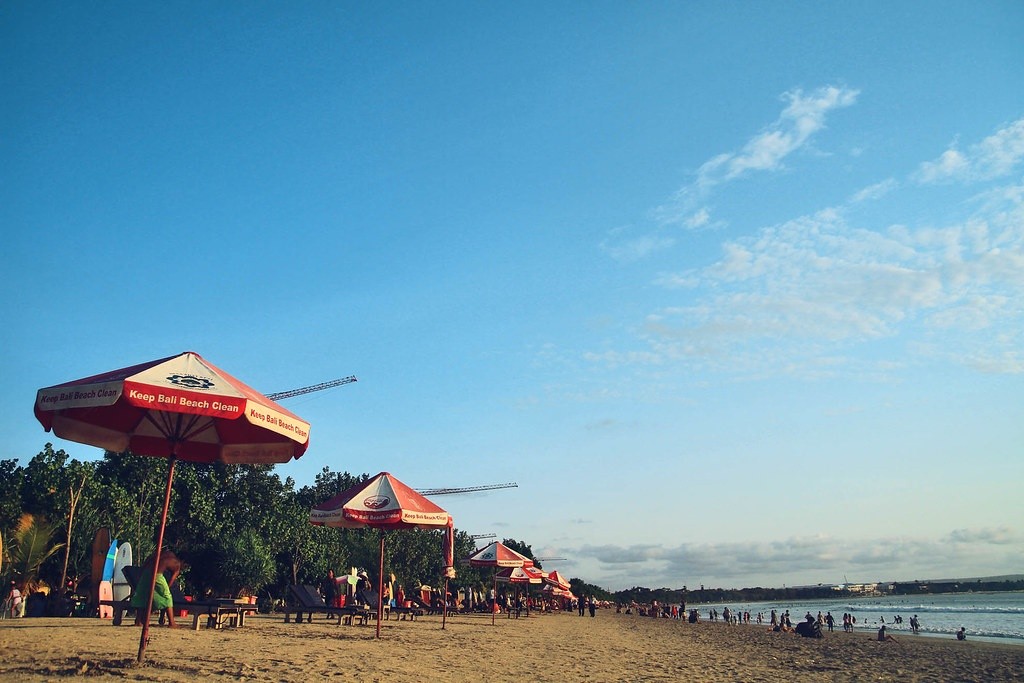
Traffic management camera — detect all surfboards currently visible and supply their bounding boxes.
[90,526,110,617]
[98,538,117,618]
[112,542,133,619]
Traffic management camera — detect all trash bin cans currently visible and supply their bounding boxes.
[245,596,258,616]
[334,594,345,608]
[404,601,411,607]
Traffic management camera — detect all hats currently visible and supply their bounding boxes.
[358,571,368,580]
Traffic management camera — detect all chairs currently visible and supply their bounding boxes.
[101,564,258,630]
[359,587,423,622]
[414,596,493,613]
[284,585,370,626]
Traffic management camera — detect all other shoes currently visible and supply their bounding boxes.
[326,616,329,619]
[329,616,334,619]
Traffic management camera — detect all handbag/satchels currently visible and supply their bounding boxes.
[773,626,781,632]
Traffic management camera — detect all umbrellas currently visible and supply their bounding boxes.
[34,352,312,662]
[492,568,540,618]
[310,472,448,637]
[442,516,454,629]
[463,541,534,624]
[526,567,577,616]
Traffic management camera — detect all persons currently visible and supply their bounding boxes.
[723,607,856,633]
[430,588,446,616]
[471,589,596,617]
[7,584,24,618]
[958,627,966,640]
[605,599,717,623]
[382,583,389,613]
[356,573,368,607]
[321,569,339,619]
[132,550,192,628]
[880,614,920,632]
[395,585,404,606]
[878,626,892,640]
